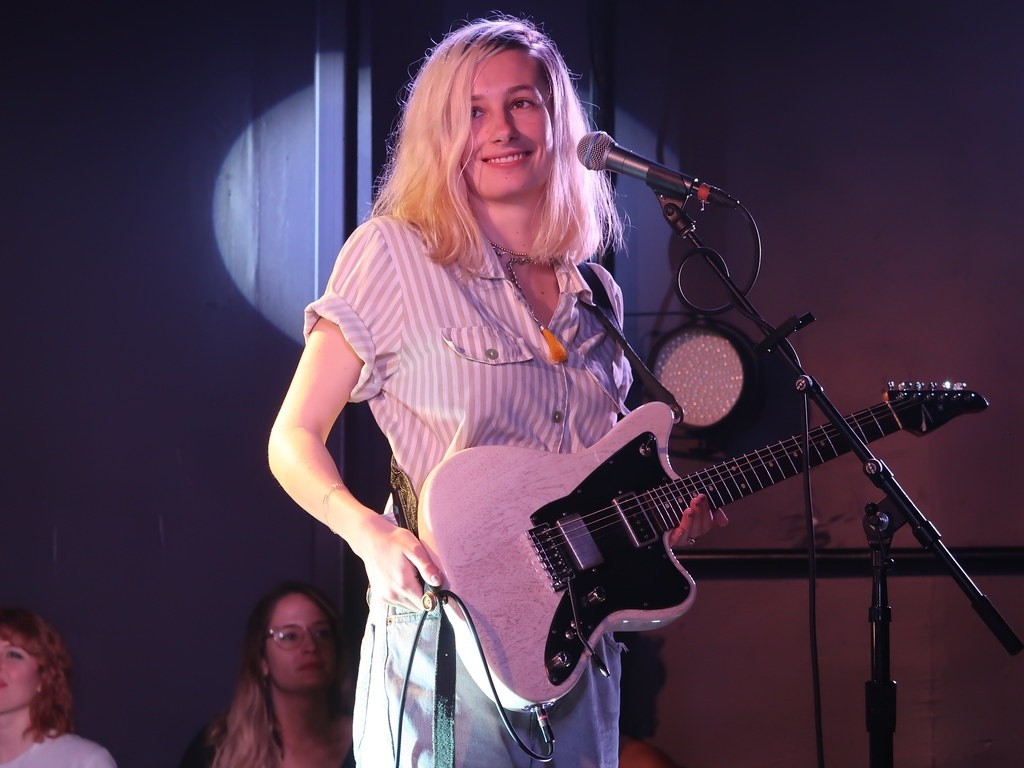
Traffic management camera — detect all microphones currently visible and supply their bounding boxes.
[577,131,740,209]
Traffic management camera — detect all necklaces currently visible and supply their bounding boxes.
[484,236,573,362]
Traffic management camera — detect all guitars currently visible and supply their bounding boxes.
[416,377,990,714]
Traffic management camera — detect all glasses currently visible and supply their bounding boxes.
[264,625,335,650]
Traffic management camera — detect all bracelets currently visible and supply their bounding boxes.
[322,483,345,528]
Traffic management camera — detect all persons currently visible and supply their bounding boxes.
[267,21,729,768]
[178,577,356,768]
[0,609,117,768]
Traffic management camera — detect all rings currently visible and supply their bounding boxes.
[688,539,696,545]
